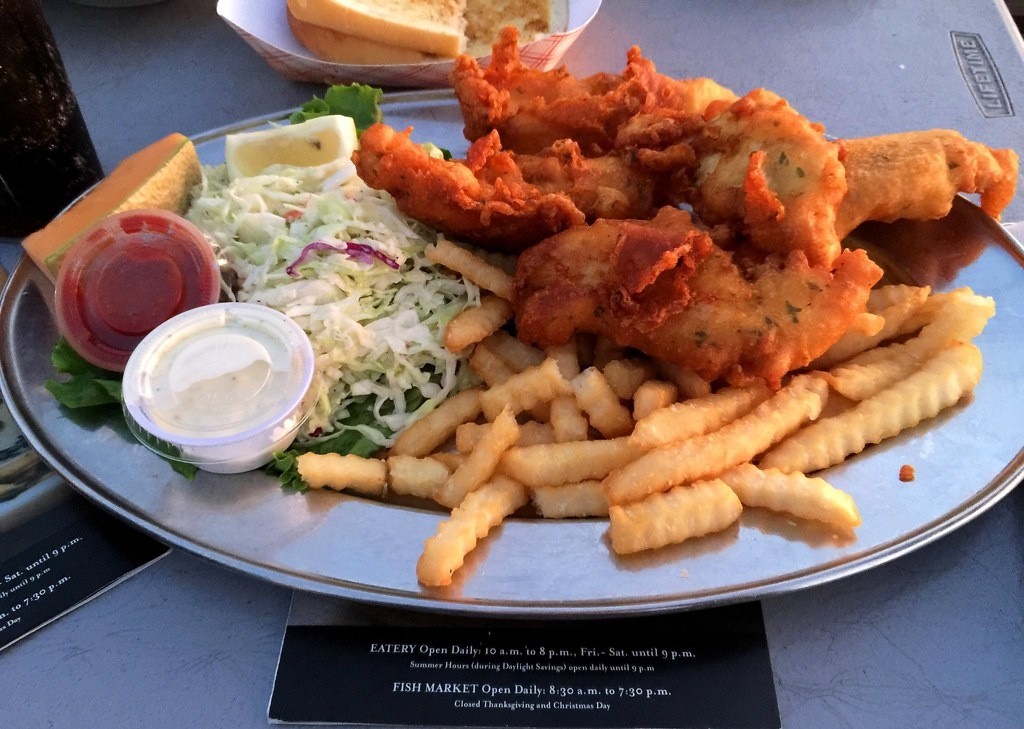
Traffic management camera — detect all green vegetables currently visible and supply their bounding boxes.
[287,82,385,135]
[43,335,384,494]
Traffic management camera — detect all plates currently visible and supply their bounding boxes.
[4,88,1024,620]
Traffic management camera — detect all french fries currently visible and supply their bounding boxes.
[295,238,997,588]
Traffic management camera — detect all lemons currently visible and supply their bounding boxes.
[224,114,357,179]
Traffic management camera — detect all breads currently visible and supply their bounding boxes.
[285,0,560,65]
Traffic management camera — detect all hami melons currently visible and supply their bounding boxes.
[19,132,206,285]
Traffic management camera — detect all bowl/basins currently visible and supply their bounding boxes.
[215,0,604,95]
[119,301,323,475]
[55,206,221,371]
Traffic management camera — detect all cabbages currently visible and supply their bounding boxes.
[184,167,481,448]
